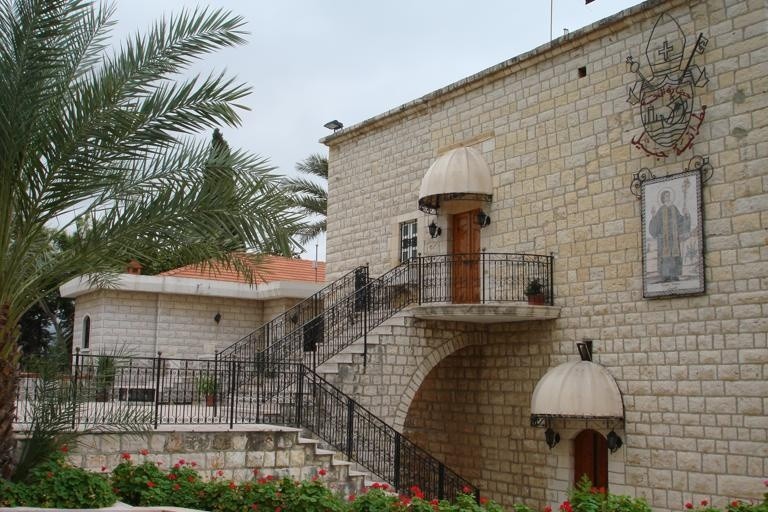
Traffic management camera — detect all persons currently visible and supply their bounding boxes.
[648,189,692,284]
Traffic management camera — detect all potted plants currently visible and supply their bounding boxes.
[524,278,543,305]
[196,374,218,407]
[95,356,115,402]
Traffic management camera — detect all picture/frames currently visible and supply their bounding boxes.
[640,169,705,298]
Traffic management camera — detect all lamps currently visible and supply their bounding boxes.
[545,428,560,450]
[428,220,440,238]
[324,120,343,133]
[606,428,622,455]
[478,210,490,228]
